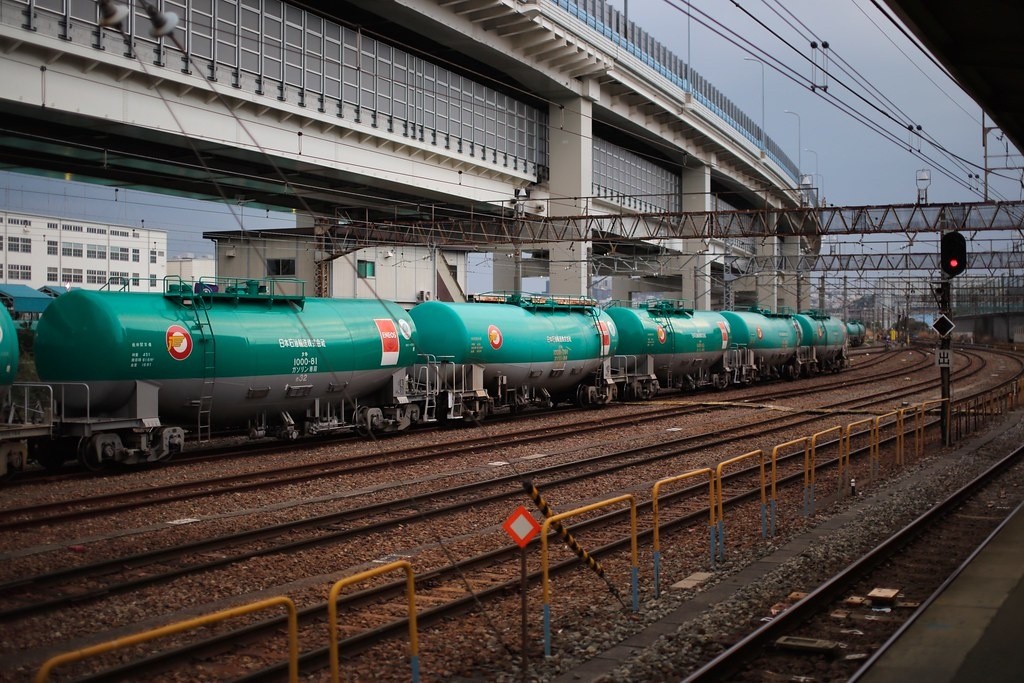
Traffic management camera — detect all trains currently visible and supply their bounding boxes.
[1,276,870,481]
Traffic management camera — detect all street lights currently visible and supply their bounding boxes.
[783,109,802,185]
[805,148,819,188]
[743,57,765,151]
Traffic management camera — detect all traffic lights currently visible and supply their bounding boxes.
[931,314,956,339]
[941,231,967,277]
